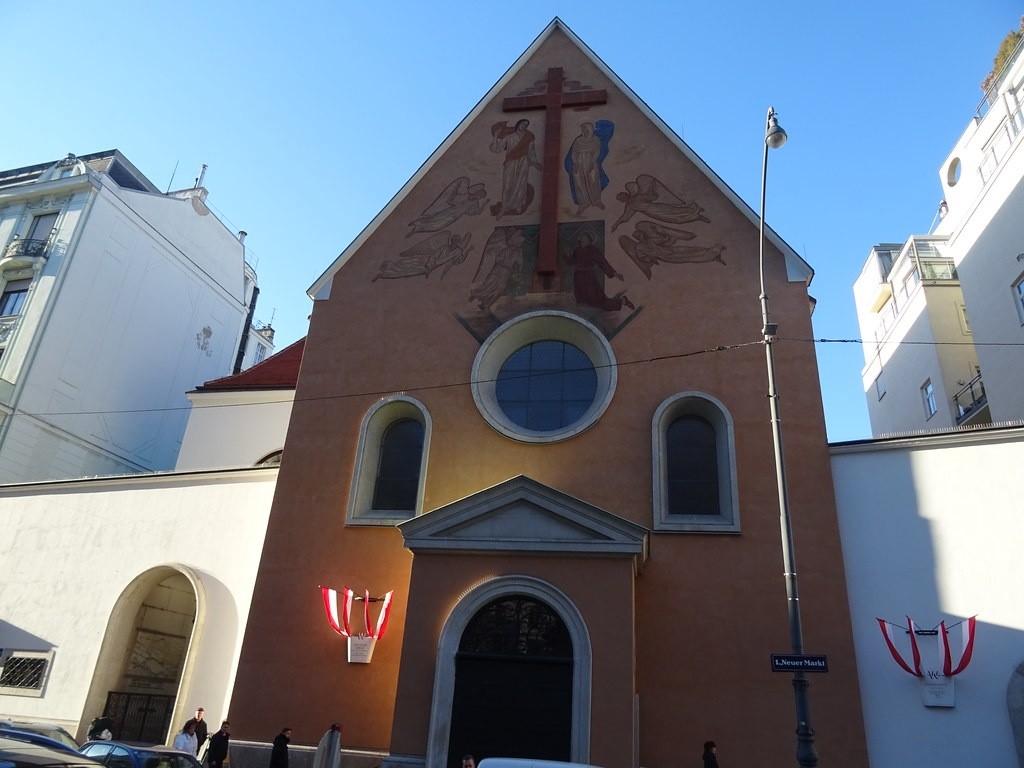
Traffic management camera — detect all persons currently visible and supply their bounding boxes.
[269,728,292,768]
[313,723,343,768]
[702,741,719,768]
[174,707,230,768]
[462,755,475,768]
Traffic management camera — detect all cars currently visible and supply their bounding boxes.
[1,719,202,767]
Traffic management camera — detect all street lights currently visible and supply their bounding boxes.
[757,104,830,767]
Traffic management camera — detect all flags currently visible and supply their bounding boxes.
[363,589,393,639]
[877,617,925,677]
[321,587,353,637]
[938,617,975,676]
